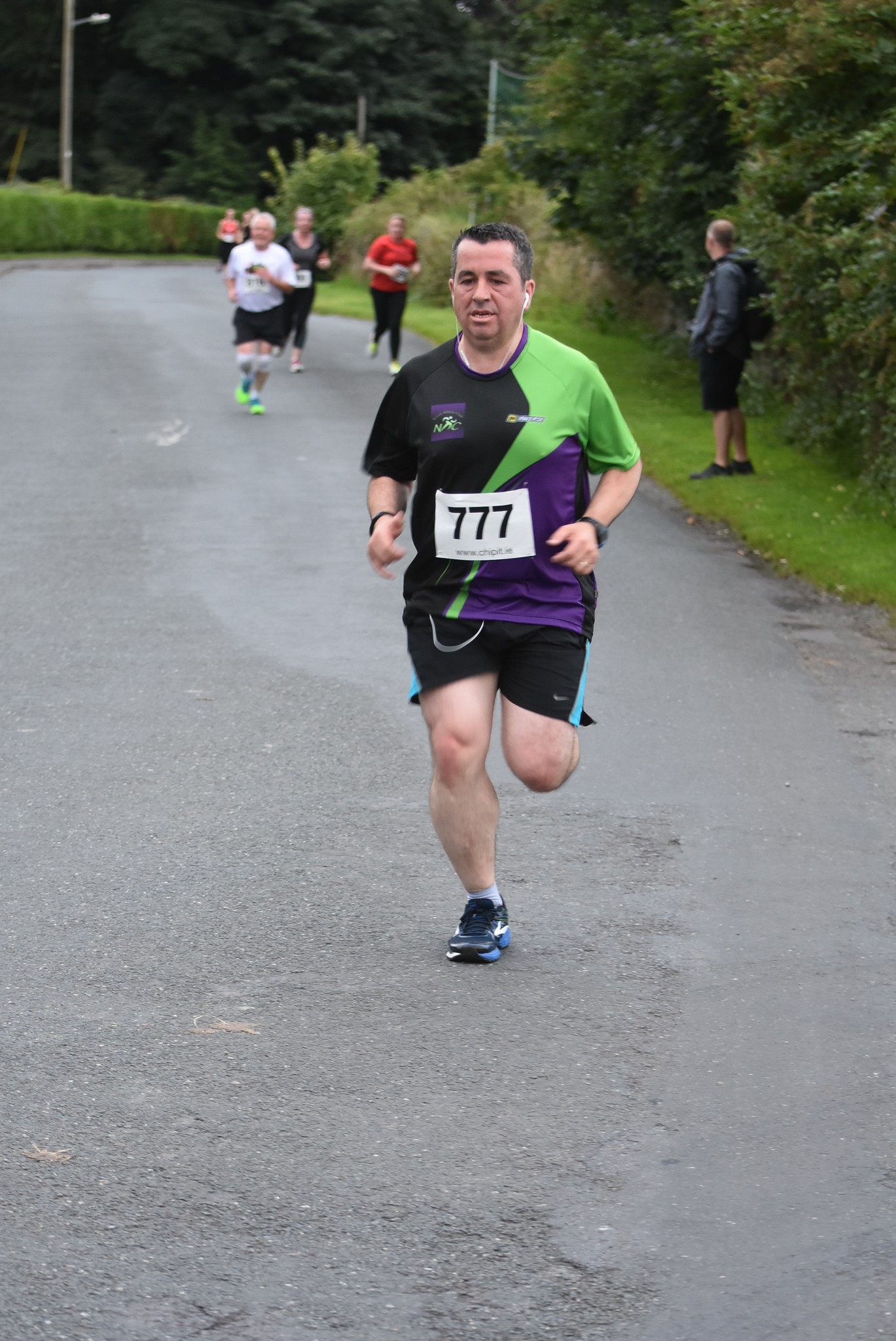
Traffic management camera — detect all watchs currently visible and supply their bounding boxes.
[576,517,608,549]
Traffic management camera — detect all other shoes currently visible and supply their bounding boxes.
[732,461,754,475]
[275,346,284,357]
[290,360,305,374]
[367,343,380,360]
[388,359,402,375]
[689,461,733,480]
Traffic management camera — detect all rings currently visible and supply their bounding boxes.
[579,562,589,567]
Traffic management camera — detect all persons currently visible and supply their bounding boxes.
[688,218,757,480]
[363,214,422,375]
[224,211,294,416]
[364,223,642,963]
[279,204,331,373]
[214,208,263,271]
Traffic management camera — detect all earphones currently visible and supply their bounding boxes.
[523,292,529,308]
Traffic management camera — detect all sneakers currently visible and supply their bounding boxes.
[235,370,254,404]
[250,399,265,414]
[445,893,511,962]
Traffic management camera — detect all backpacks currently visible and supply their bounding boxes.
[709,260,773,343]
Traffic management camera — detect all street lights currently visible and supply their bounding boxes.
[59,13,111,192]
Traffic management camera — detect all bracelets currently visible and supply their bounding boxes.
[369,511,395,536]
